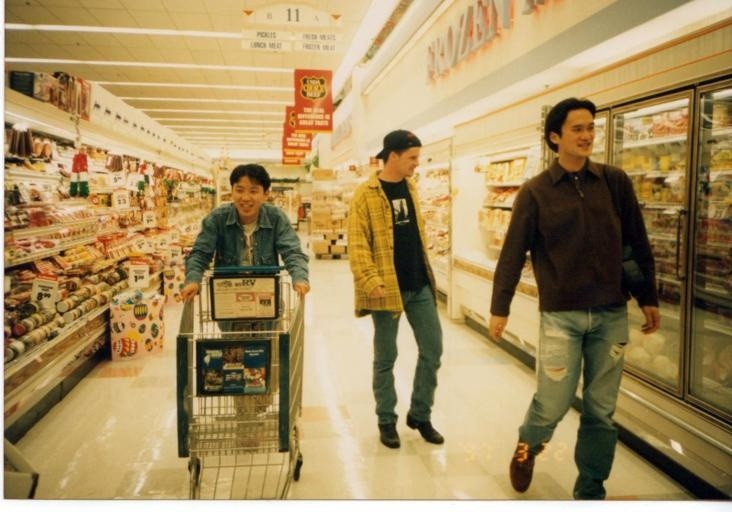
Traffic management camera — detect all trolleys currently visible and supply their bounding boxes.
[177,266,305,501]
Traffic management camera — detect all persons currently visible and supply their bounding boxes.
[348,129,443,448]
[490,97,660,501]
[180,164,309,451]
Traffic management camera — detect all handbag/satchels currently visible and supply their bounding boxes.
[602,164,647,296]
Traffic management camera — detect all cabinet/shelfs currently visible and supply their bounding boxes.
[610,81,695,397]
[450,127,545,358]
[408,160,451,301]
[687,83,731,425]
[545,110,609,167]
[6,95,216,438]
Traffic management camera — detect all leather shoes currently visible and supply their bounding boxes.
[510,436,544,493]
[378,423,400,448]
[407,414,443,444]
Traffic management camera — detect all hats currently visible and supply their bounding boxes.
[375,129,422,158]
